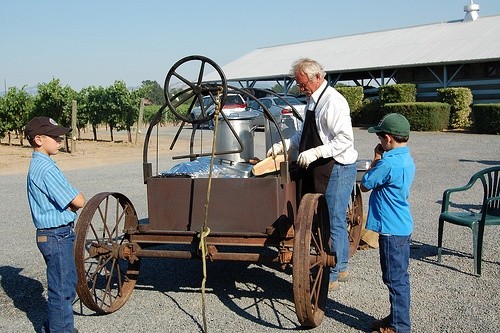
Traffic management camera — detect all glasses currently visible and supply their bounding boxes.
[296,79,310,88]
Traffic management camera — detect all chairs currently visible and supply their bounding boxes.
[437,166,500,277]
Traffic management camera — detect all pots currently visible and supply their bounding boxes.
[264,110,304,160]
[212,109,258,163]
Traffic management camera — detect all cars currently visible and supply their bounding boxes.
[250,95,306,131]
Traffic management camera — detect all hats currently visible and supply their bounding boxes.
[367,112,410,136]
[24,117,73,140]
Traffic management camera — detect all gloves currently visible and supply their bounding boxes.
[267,138,293,159]
[296,147,323,169]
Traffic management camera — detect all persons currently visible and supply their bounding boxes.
[267,58,358,290]
[360,113,415,333]
[24,115,86,333]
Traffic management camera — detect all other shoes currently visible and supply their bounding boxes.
[328,280,339,291]
[370,314,397,333]
[41,322,79,333]
[338,271,350,282]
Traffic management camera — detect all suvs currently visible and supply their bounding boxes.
[189,93,251,130]
